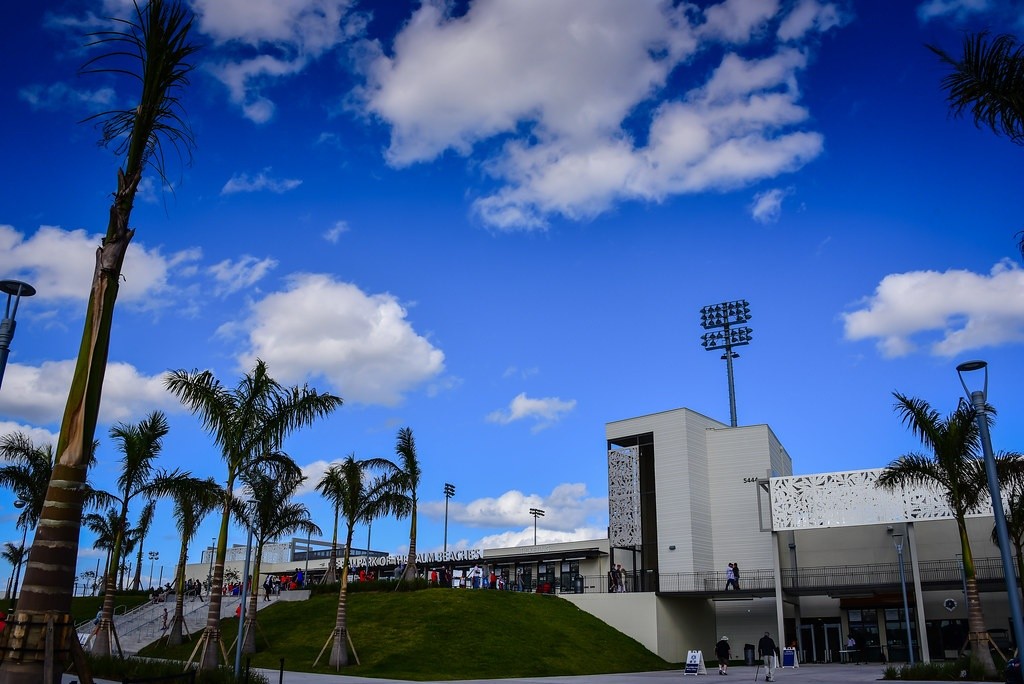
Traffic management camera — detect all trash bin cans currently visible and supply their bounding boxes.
[574,574,585,593]
[744,644,755,666]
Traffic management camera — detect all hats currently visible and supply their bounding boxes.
[720,636,729,641]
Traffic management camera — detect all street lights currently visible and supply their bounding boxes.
[443,482,456,560]
[148,550,159,590]
[0,278,36,390]
[891,533,915,665]
[365,518,373,575]
[206,537,218,598]
[699,299,753,426]
[955,358,1024,681]
[8,492,33,614]
[529,508,545,545]
[234,498,262,677]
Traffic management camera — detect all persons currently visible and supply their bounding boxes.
[609,564,626,592]
[94,606,104,625]
[274,568,303,599]
[759,632,778,682]
[724,563,739,590]
[473,561,533,592]
[149,583,172,604]
[431,566,449,589]
[235,602,247,617]
[221,577,251,595]
[360,568,376,582]
[190,579,204,602]
[264,576,273,602]
[459,573,466,588]
[714,636,731,676]
[393,564,401,580]
[845,634,855,663]
[161,608,169,629]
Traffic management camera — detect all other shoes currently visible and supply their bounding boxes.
[724,672,727,675]
[719,670,723,675]
[770,679,776,682]
[765,675,769,682]
